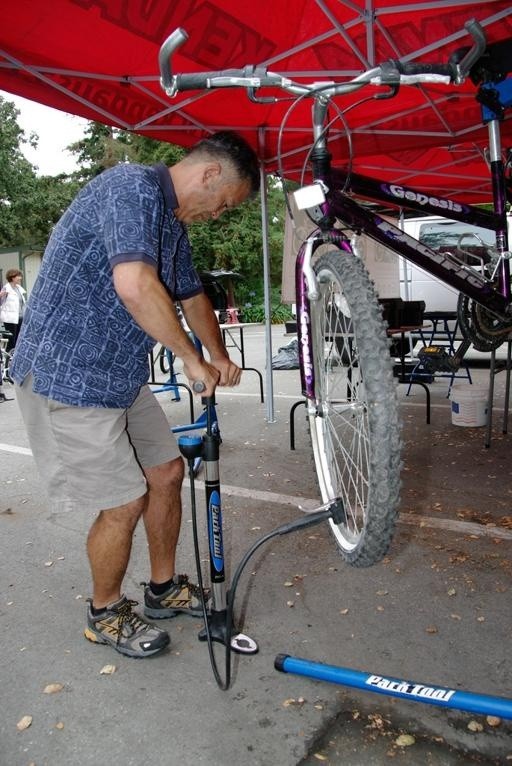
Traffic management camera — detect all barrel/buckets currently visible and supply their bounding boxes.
[449,384,490,428]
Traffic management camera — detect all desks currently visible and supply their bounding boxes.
[145,319,264,425]
[281,309,473,452]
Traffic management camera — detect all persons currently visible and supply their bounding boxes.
[0,269,27,375]
[5,128,262,662]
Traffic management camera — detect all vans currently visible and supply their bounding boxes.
[175,268,249,332]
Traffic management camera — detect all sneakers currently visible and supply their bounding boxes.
[140,575,211,620]
[84,593,170,658]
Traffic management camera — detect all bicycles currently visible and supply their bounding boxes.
[158,341,178,374]
[152,14,512,573]
[0,330,17,385]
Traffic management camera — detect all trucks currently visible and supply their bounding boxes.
[281,213,512,369]
